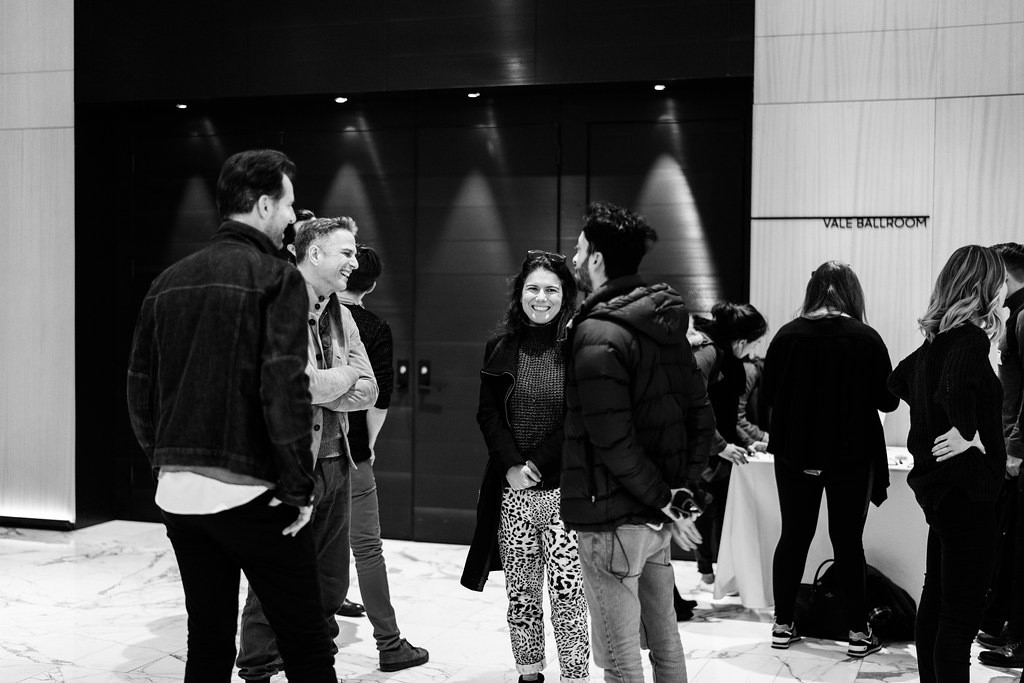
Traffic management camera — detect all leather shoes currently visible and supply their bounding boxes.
[977,632,1012,650]
[336,599,365,616]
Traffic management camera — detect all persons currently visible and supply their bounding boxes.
[886,245,1007,682]
[127,151,339,682]
[765,261,901,656]
[461,250,591,682]
[693,356,766,598]
[558,201,716,682]
[675,301,767,625]
[976,243,1024,682]
[233,215,378,682]
[337,245,430,683]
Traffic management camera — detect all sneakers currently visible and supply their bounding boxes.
[379,637,429,672]
[772,620,802,649]
[847,622,882,657]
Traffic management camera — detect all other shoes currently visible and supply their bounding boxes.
[518,672,544,683]
[702,573,715,584]
[977,640,1024,669]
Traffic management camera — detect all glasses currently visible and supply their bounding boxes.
[527,249,567,265]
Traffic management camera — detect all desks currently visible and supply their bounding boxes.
[708,441,936,620]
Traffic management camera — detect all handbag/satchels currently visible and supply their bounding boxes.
[801,557,917,645]
